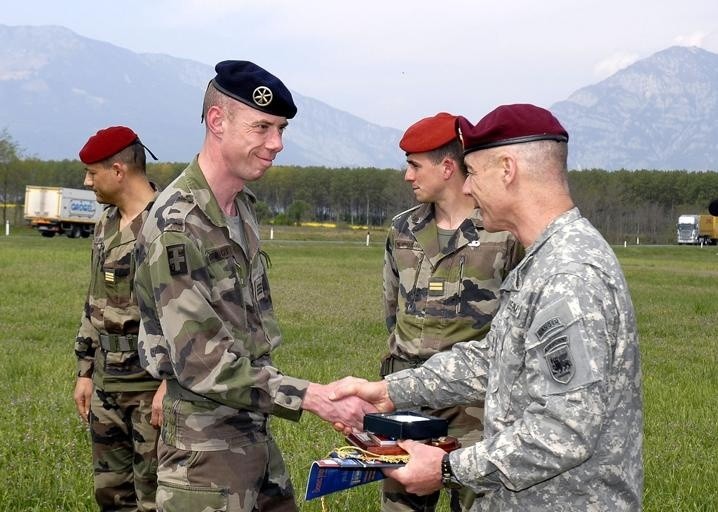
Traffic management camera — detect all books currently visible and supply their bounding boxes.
[305,445,410,502]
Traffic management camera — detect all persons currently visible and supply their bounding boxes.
[377,112,527,512]
[328,103,647,512]
[133,59,382,512]
[71,126,166,510]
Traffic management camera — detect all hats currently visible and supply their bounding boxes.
[466,103,569,152]
[213,59,297,120]
[399,111,466,152]
[79,126,136,163]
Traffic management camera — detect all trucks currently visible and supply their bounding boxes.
[24,185,110,238]
[676,214,718,245]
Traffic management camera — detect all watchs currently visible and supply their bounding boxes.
[441,452,464,492]
